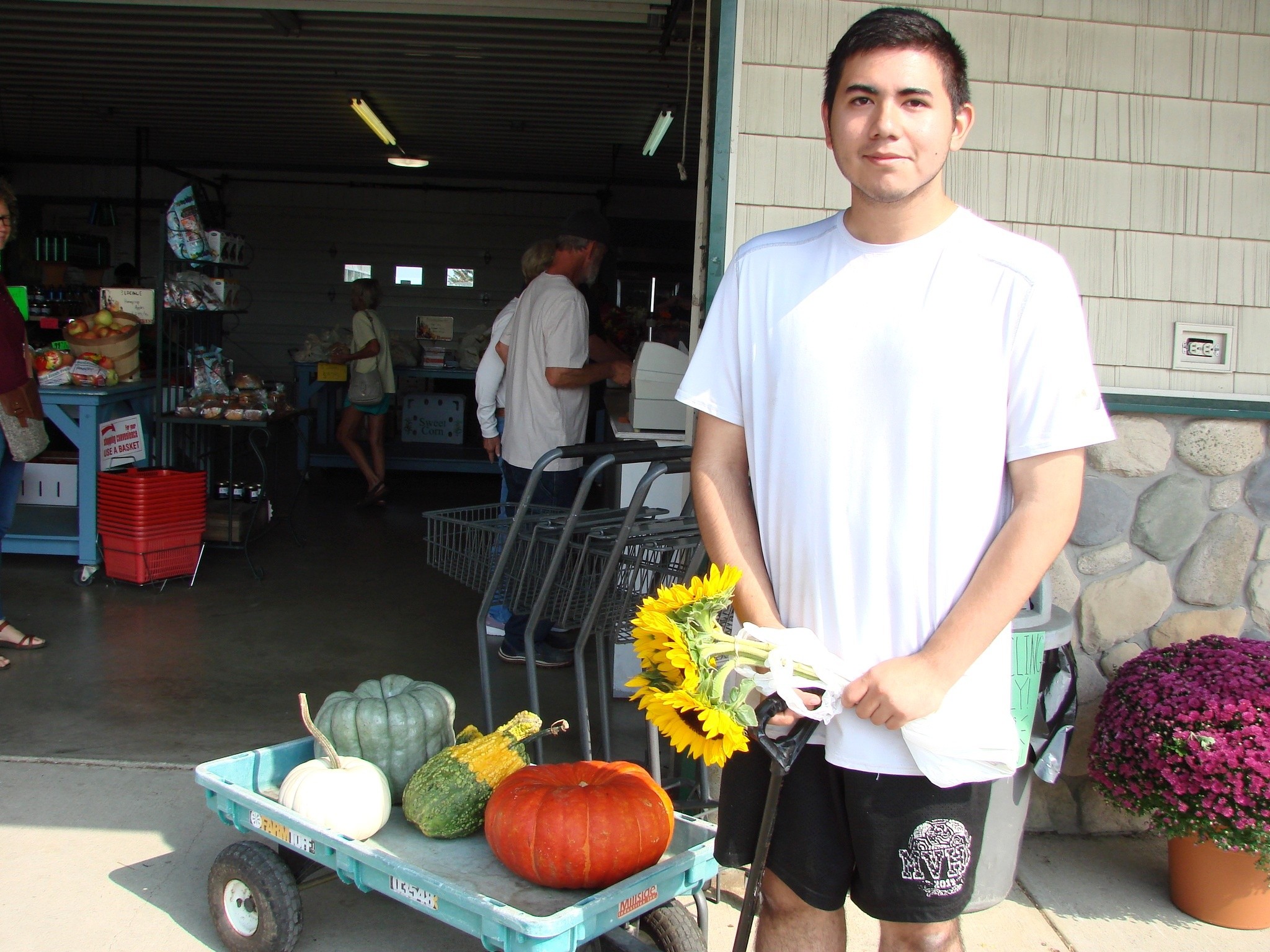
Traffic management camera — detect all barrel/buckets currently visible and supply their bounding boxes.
[62,311,142,382]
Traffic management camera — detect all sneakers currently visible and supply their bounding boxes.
[498,637,575,669]
[485,604,512,636]
[541,630,578,652]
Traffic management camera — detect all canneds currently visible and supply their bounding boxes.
[30,291,51,316]
[215,481,266,503]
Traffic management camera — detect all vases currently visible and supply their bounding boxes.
[1167,825,1270,930]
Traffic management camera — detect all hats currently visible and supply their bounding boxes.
[556,209,622,248]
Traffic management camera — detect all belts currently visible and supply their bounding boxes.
[495,407,505,417]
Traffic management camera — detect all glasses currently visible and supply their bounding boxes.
[0,215,17,226]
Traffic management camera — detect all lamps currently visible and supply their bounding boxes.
[348,91,397,145]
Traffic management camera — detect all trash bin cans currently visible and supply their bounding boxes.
[958,572,1074,916]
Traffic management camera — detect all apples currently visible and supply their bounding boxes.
[34,349,118,386]
[67,308,134,340]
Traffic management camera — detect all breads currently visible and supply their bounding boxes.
[177,373,289,421]
[293,324,421,367]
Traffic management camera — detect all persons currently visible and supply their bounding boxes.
[493,218,630,668]
[0,191,48,671]
[473,238,615,637]
[675,5,1118,952]
[342,279,396,505]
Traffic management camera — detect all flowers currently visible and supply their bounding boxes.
[624,563,822,766]
[1087,634,1270,887]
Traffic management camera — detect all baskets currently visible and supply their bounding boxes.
[98,466,208,584]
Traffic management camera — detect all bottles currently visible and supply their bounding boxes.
[215,479,265,505]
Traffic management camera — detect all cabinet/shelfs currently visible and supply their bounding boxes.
[290,361,502,476]
[608,408,694,595]
[156,205,318,580]
[1,379,156,588]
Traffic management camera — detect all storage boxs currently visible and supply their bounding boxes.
[401,392,467,444]
[317,361,347,381]
[17,451,80,506]
[203,228,246,310]
[423,347,455,369]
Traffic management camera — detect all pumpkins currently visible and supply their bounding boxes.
[484,761,674,889]
[277,675,570,841]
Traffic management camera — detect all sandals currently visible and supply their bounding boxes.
[360,481,387,509]
[357,494,386,506]
[0,655,11,670]
[0,621,46,649]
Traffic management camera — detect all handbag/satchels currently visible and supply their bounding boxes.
[347,368,383,407]
[731,620,1018,788]
[0,380,50,462]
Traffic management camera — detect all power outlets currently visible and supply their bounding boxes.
[1173,322,1235,373]
